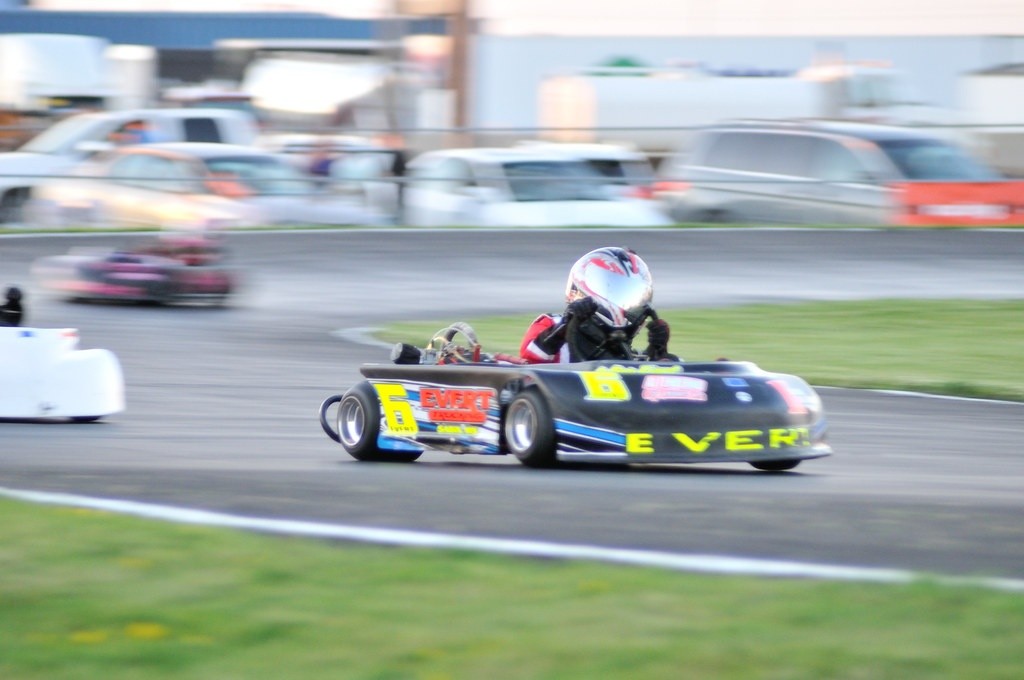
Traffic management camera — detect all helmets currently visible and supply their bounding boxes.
[565,246,653,340]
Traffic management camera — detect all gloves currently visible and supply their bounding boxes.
[645,319,670,349]
[562,296,597,326]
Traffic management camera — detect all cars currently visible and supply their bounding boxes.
[1,108,1023,229]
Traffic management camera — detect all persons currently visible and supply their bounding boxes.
[519,245,673,365]
